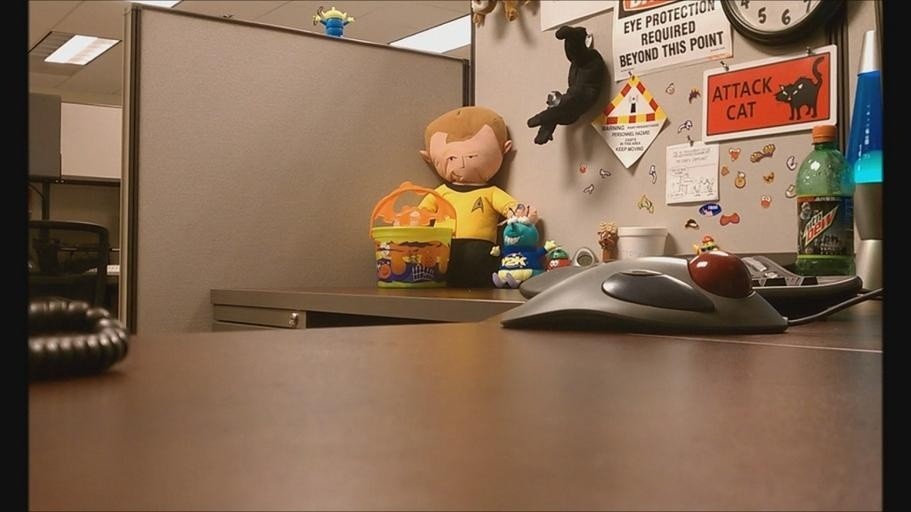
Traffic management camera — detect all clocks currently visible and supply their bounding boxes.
[722,0,843,48]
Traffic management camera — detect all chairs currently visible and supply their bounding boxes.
[30,219,109,308]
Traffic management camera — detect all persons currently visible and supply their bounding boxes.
[389,104,541,290]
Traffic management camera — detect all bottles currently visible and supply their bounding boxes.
[798,126,856,275]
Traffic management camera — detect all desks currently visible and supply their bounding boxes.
[25,287,883,512]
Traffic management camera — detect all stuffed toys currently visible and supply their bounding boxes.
[471,0,531,27]
[526,24,606,148]
[540,240,574,271]
[488,207,546,289]
